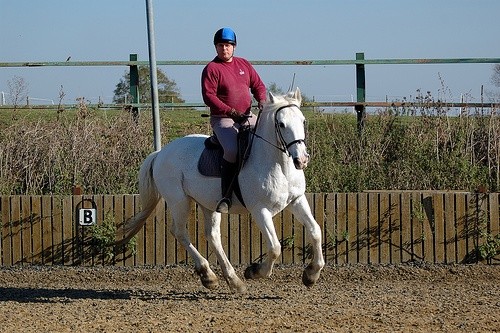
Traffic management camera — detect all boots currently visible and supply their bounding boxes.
[216,158,238,214]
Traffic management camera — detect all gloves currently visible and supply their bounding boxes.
[258,99,266,110]
[228,108,248,125]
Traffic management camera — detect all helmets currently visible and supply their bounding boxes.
[213,28,237,46]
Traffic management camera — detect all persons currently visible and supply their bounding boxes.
[201,28,267,213]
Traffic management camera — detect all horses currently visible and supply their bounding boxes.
[105,87,325,295]
